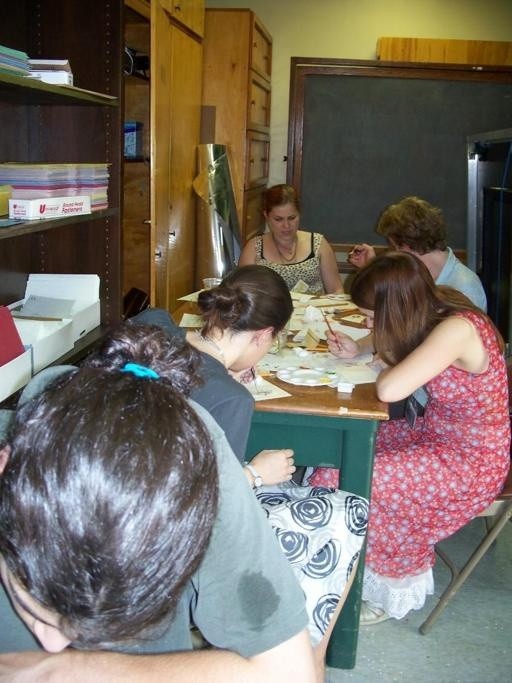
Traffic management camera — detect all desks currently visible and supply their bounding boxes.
[169,290,390,669]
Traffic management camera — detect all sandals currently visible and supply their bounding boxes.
[360,601,394,625]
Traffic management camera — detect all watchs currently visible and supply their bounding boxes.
[247,464,264,488]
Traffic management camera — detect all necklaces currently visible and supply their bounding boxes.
[195,331,229,369]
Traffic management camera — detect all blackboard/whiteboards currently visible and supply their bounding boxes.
[285,56,512,253]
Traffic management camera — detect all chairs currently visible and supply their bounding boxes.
[419,486,512,635]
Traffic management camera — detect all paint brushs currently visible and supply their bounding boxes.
[322,310,344,353]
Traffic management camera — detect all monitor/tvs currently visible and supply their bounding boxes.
[481,184,512,343]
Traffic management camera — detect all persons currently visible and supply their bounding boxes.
[345,193,488,315]
[236,182,346,297]
[94,261,375,683]
[1,314,324,683]
[323,247,510,627]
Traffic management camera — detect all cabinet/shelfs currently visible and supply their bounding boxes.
[0,0,126,410]
[194,8,273,292]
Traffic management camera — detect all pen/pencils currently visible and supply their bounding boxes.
[251,368,259,392]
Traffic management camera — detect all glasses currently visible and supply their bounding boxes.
[269,330,280,353]
